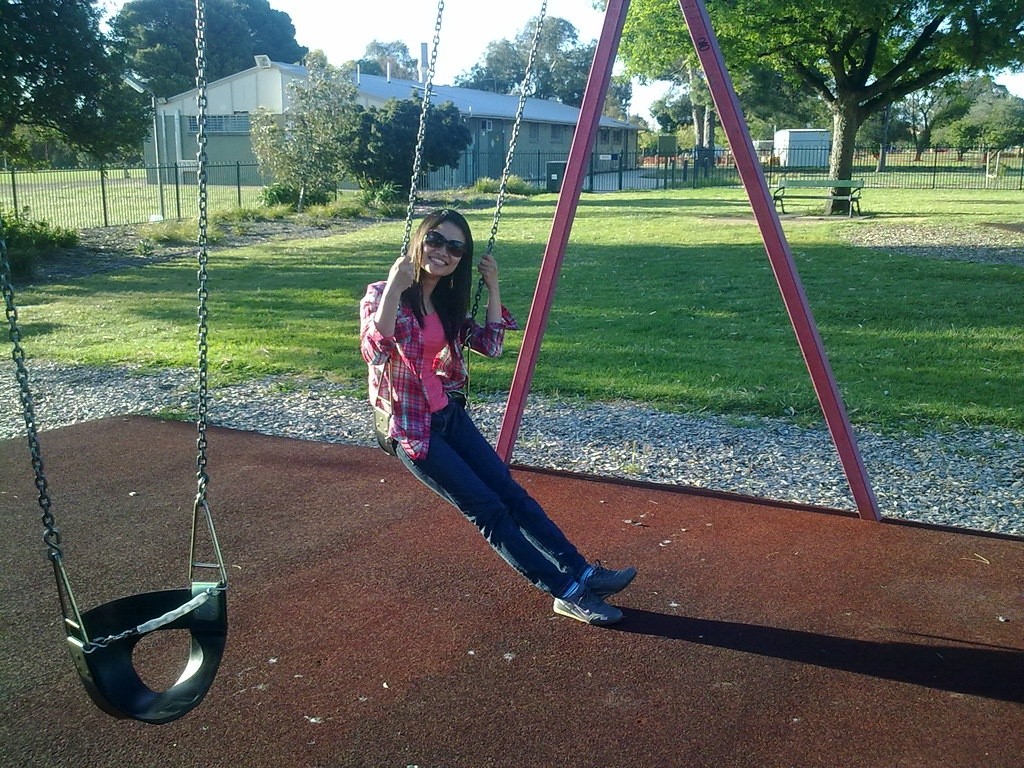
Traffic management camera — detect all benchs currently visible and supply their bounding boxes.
[771,179,864,218]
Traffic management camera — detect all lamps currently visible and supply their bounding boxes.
[157,96,168,105]
[254,54,271,69]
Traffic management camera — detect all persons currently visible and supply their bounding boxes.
[360,210,636,624]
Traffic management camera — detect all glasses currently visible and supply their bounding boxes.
[423,231,466,257]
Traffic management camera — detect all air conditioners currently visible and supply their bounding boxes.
[482,119,492,131]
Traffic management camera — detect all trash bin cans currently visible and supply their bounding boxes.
[546,161,567,193]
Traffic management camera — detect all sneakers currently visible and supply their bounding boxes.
[586,559,637,600]
[553,582,623,625]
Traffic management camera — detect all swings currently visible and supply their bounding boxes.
[0,0,236,726]
[372,0,549,459]
